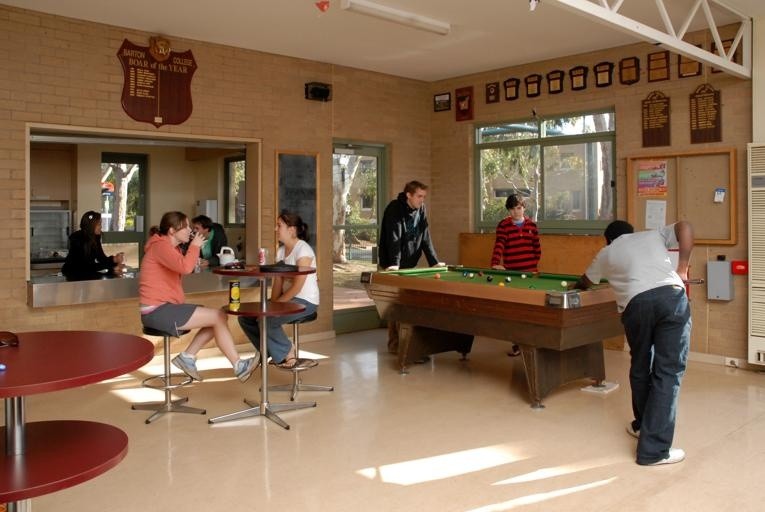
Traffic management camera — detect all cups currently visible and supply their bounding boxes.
[189,229,207,249]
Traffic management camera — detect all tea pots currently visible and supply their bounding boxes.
[216,246,235,265]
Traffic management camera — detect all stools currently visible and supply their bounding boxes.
[129,327,206,426]
[256,310,334,403]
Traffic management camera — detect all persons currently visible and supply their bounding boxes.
[65,271,123,281]
[137,210,261,383]
[192,265,218,272]
[236,211,320,369]
[378,180,448,357]
[183,214,229,269]
[60,210,124,274]
[492,192,542,358]
[566,219,695,467]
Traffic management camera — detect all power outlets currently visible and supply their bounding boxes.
[725,357,739,368]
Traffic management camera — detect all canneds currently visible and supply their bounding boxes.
[258,247,270,266]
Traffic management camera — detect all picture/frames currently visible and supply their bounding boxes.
[433,91,451,112]
[455,85,475,122]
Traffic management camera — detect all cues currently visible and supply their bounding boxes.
[567,279,704,286]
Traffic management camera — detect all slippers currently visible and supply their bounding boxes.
[278,357,304,369]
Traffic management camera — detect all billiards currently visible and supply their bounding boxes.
[561,281,567,287]
[434,274,440,279]
[521,274,527,279]
[498,282,504,287]
[487,275,493,282]
[505,277,511,282]
[462,271,483,278]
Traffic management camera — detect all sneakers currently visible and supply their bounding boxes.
[234,350,261,383]
[627,423,641,439]
[648,448,685,465]
[510,345,520,355]
[171,352,203,382]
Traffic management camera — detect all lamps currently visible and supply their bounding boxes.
[340,0,451,35]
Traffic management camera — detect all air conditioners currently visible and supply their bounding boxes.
[745,141,765,368]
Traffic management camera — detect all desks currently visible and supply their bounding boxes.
[358,264,632,413]
[0,328,156,512]
[206,259,320,431]
